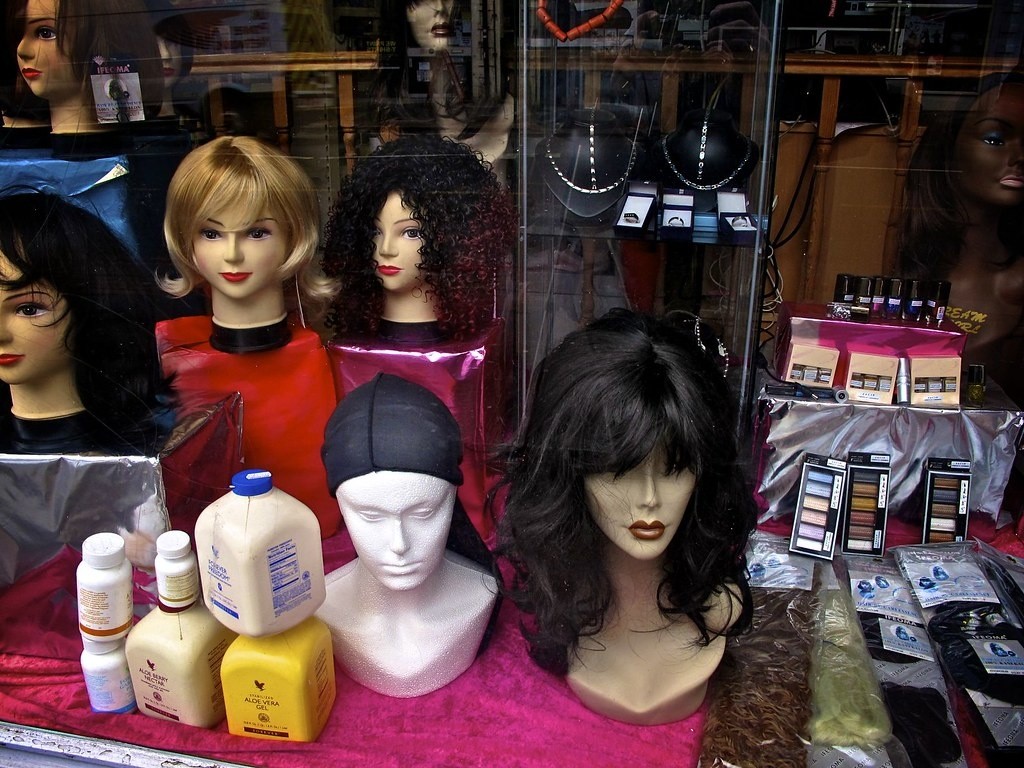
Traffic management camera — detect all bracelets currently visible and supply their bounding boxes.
[667,217,684,227]
[731,215,748,227]
[623,212,641,224]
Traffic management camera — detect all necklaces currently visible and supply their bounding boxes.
[662,105,753,191]
[546,108,637,194]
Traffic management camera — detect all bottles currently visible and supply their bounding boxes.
[154,529,200,609]
[193,468,325,639]
[75,533,134,641]
[124,597,239,729]
[220,615,336,741]
[81,635,138,715]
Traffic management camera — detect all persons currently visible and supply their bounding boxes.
[894,70,1024,392]
[151,133,341,354]
[146,0,197,133]
[488,304,756,727]
[314,369,506,700]
[320,136,523,343]
[0,172,185,456]
[608,0,773,128]
[362,0,515,196]
[0,0,167,161]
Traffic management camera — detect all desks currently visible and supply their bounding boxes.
[0,443,1023,768]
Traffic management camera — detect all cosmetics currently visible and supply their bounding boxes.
[789,362,957,394]
[790,469,836,553]
[963,363,987,409]
[929,473,960,544]
[847,469,880,551]
[826,274,952,327]
